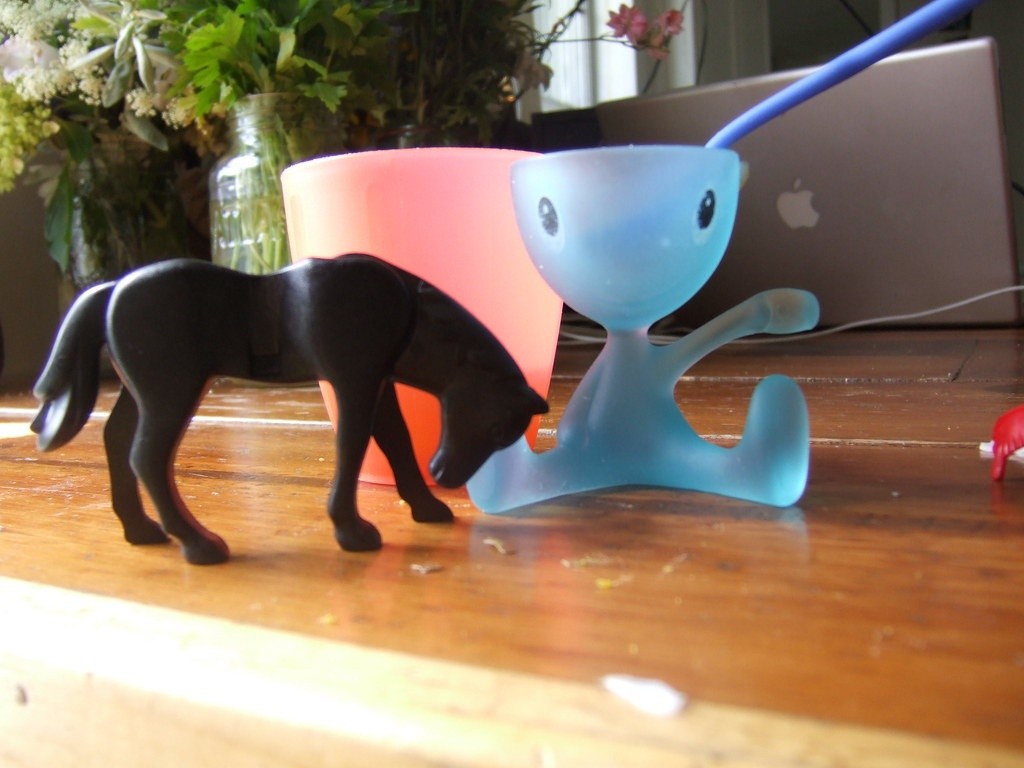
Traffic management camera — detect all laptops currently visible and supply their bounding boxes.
[597,36,1024,328]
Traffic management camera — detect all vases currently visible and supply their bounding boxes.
[52,141,188,382]
[209,91,353,383]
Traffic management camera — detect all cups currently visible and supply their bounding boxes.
[281,149,564,485]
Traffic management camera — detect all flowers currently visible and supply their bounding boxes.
[0,0,688,259]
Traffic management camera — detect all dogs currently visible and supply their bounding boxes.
[29,251,550,566]
